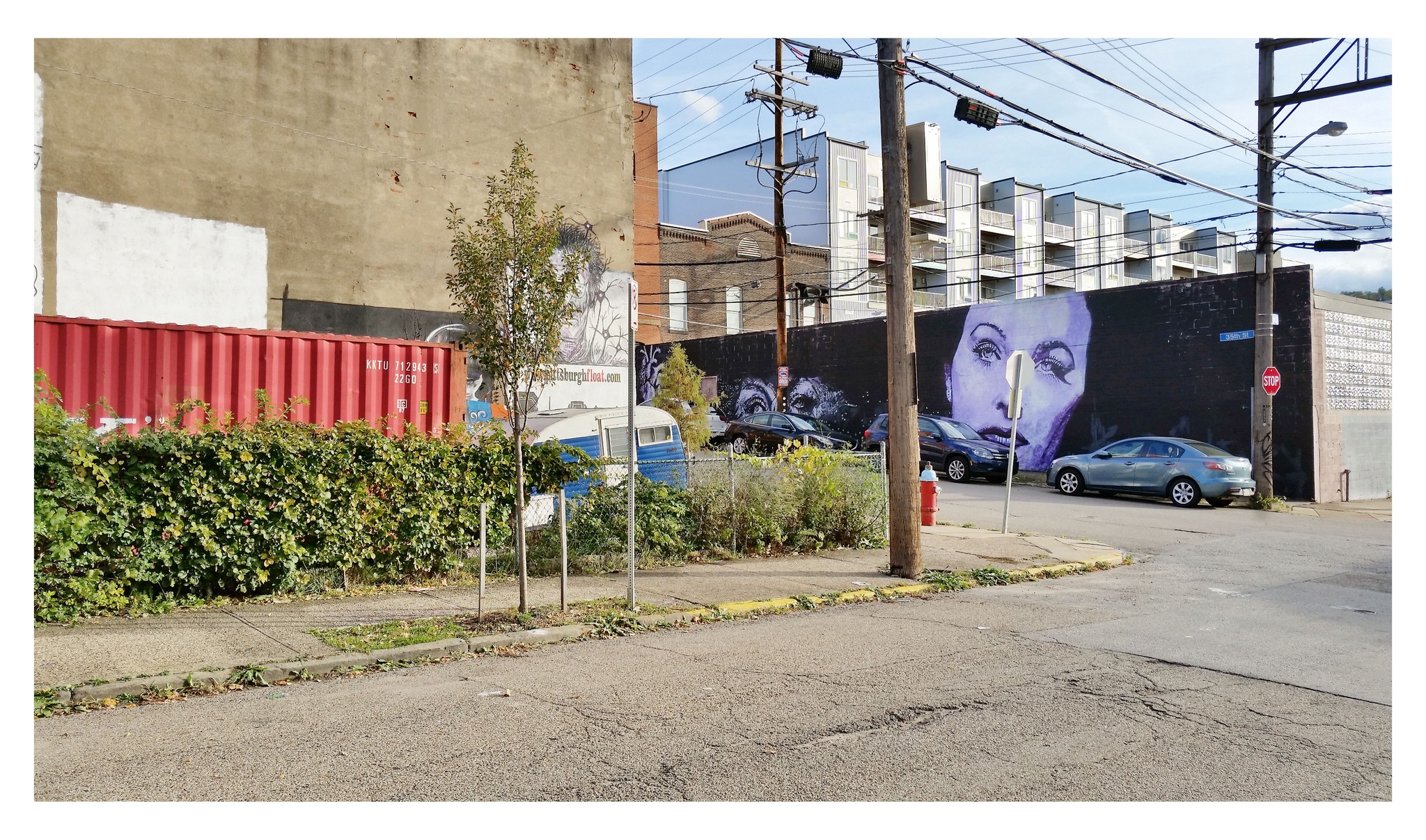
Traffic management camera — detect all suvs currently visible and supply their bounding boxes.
[637,396,727,452]
[859,412,1020,486]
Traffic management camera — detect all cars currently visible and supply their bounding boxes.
[722,410,860,458]
[1043,435,1257,510]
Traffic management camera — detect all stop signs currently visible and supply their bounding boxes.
[1260,365,1281,396]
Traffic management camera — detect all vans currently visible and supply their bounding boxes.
[458,406,690,552]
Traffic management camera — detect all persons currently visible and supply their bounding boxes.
[940,294,1104,473]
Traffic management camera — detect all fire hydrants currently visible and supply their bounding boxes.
[917,458,942,526]
